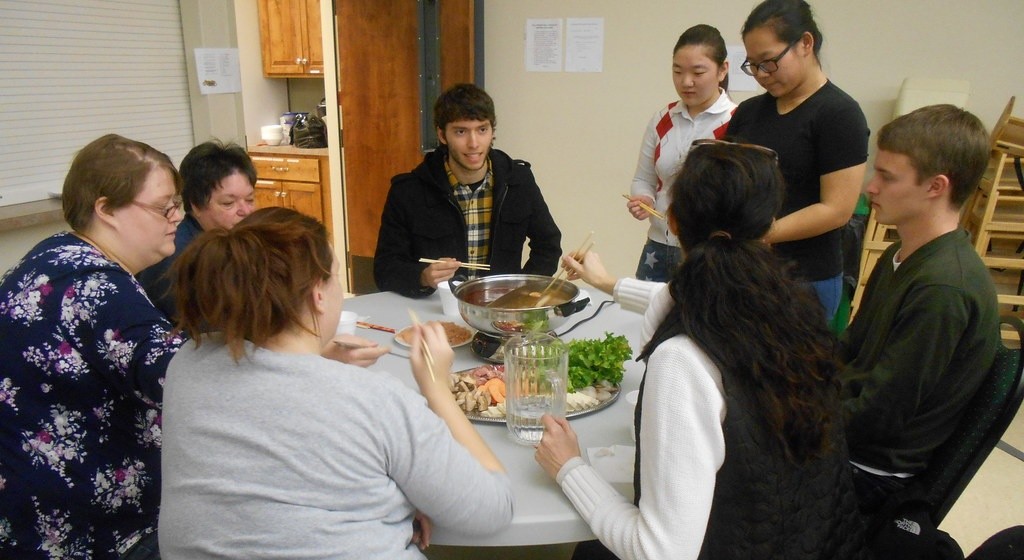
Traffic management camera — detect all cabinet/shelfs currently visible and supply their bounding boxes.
[248,152,334,242]
[256,0,323,80]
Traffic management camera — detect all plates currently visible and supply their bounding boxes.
[446,364,622,423]
[394,321,475,350]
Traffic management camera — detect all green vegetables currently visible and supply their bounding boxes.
[540,331,634,393]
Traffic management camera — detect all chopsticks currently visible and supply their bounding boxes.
[540,231,595,296]
[418,258,490,271]
[406,306,436,384]
[622,194,664,221]
[332,340,410,360]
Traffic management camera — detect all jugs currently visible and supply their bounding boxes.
[503,333,569,446]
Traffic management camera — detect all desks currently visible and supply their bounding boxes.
[324,285,644,548]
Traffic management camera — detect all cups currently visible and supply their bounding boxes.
[437,281,466,316]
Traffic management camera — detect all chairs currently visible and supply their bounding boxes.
[848,96,1024,342]
[930,312,1024,560]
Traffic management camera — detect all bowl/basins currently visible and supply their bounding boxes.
[260,112,309,146]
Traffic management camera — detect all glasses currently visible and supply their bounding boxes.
[688,139,779,167]
[130,200,183,218]
[741,45,790,76]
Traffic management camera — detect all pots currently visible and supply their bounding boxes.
[447,273,590,361]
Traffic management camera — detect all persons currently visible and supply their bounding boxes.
[133,142,258,316]
[160,206,519,560]
[536,138,867,560]
[722,0,870,321]
[0,133,184,560]
[627,24,738,283]
[373,83,562,298]
[833,105,999,514]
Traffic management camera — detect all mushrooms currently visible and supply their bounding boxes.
[450,372,489,413]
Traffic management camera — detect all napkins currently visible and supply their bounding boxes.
[584,444,635,483]
